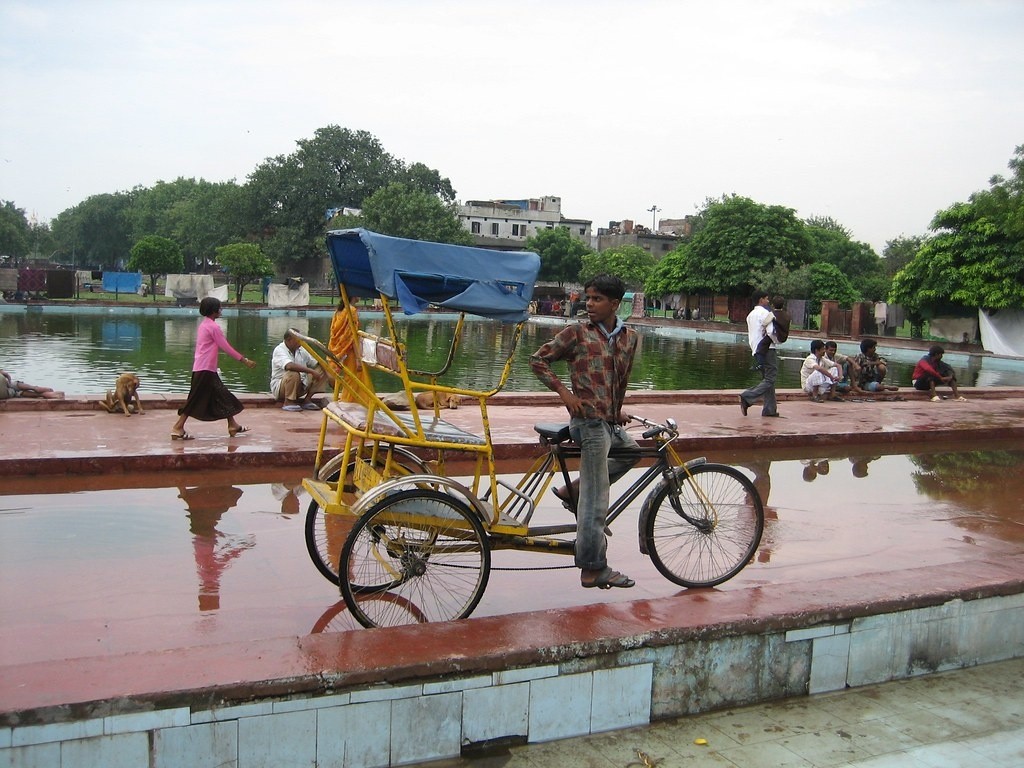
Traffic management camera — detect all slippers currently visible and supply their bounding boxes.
[581,566,636,588]
[952,396,968,403]
[302,403,320,410]
[552,487,578,506]
[282,403,303,412]
[929,396,942,402]
[827,396,844,402]
[811,397,824,403]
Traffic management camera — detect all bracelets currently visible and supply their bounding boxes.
[245,358,248,362]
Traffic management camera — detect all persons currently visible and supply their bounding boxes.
[272,481,308,520]
[528,273,643,588]
[326,296,375,403]
[174,476,257,612]
[0,367,66,399]
[848,339,899,391]
[802,454,881,481]
[269,328,327,412]
[911,346,969,403]
[170,297,257,439]
[691,307,698,320]
[915,453,936,470]
[736,461,778,565]
[547,294,562,316]
[799,339,862,402]
[738,290,791,417]
[676,307,685,319]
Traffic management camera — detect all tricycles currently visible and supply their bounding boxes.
[286,225,765,629]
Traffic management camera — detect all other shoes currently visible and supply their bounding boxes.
[764,412,779,417]
[749,364,763,371]
[739,395,750,416]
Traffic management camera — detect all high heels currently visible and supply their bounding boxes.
[171,432,196,441]
[229,425,250,435]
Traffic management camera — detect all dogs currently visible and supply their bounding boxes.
[380,389,416,411]
[414,391,462,409]
[99,374,145,417]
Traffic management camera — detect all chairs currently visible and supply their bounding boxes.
[288,328,489,515]
